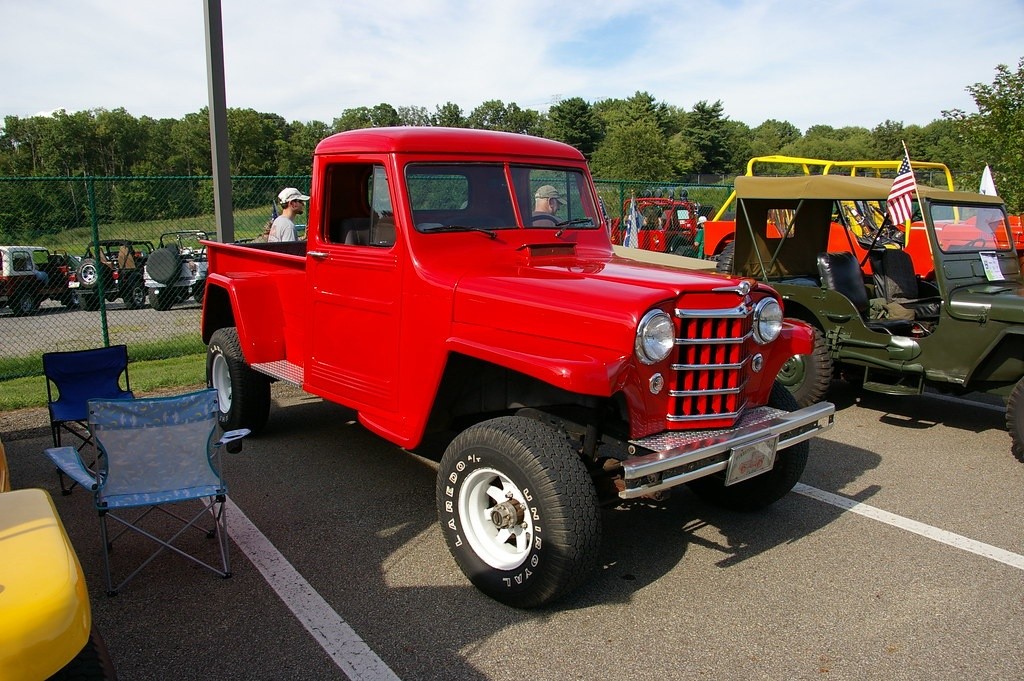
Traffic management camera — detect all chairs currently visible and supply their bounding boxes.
[817,251,914,336]
[85,388,232,599]
[338,217,397,247]
[869,248,943,321]
[42,345,135,494]
[442,214,507,230]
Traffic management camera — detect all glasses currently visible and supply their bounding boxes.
[294,200,303,203]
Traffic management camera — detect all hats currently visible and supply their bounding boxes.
[278,188,310,204]
[697,216,708,225]
[535,185,568,204]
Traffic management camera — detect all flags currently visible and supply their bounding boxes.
[272,201,278,220]
[623,195,644,248]
[599,195,611,236]
[886,154,917,226]
[976,167,1005,235]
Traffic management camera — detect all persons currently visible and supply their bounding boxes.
[694,216,708,260]
[531,185,568,227]
[250,218,274,243]
[267,187,310,242]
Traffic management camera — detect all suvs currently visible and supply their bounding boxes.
[0,230,209,317]
[199,127,836,609]
[606,188,715,258]
[704,155,1024,463]
